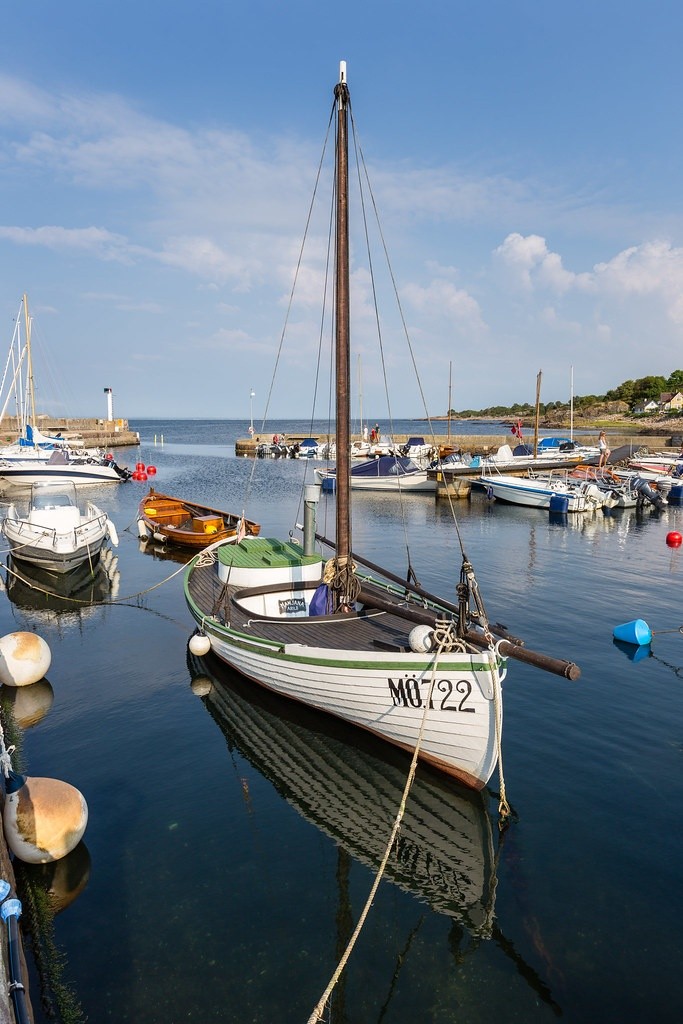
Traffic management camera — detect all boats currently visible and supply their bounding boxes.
[5,554,120,627]
[314,456,438,490]
[462,451,683,514]
[257,361,580,459]
[1,480,119,574]
[136,486,262,549]
[140,535,196,564]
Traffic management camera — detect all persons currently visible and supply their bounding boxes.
[273,434,279,444]
[598,430,611,468]
[364,425,368,442]
[375,423,380,441]
[279,433,285,445]
[370,427,376,440]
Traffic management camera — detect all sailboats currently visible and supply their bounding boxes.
[0,293,133,483]
[182,61,581,792]
[187,626,563,1024]
